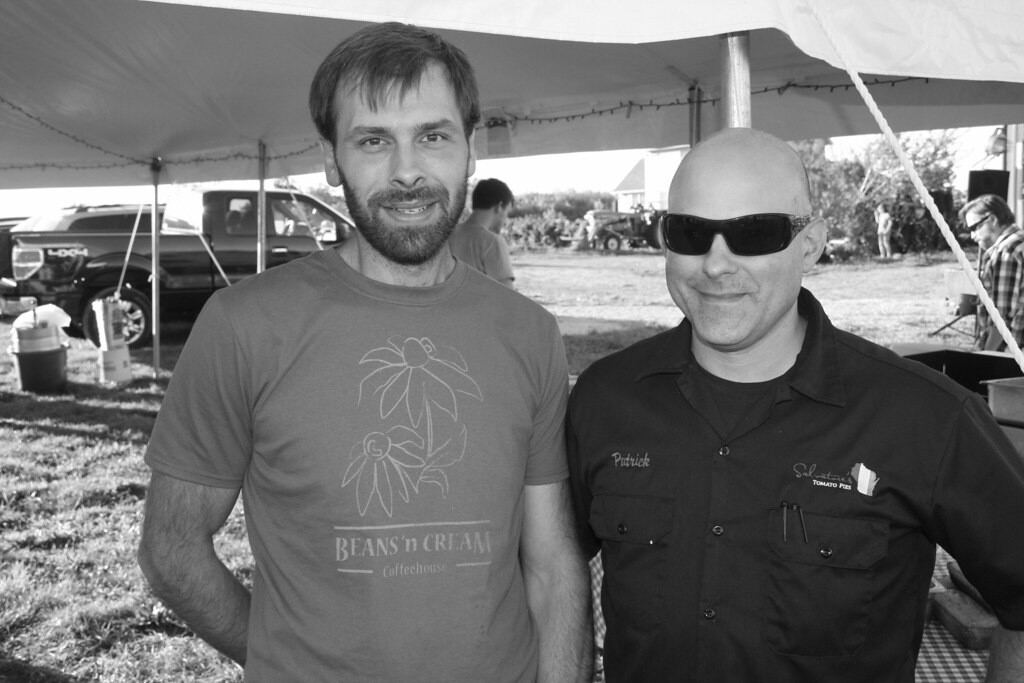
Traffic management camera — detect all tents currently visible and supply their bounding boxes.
[0,0,1024,382]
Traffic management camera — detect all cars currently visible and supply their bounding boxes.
[555,207,664,254]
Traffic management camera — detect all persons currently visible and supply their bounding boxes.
[873,204,892,258]
[170,21,595,683]
[446,179,516,290]
[565,128,1024,683]
[959,193,1024,353]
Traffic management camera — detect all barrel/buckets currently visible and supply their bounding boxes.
[6,344,71,395]
[98,346,131,384]
[92,301,128,349]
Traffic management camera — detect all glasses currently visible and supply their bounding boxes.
[660,211,806,256]
[969,217,986,234]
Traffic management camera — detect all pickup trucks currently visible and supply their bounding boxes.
[0,183,359,353]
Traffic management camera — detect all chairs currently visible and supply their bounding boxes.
[226,210,241,234]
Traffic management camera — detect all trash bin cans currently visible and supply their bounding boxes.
[7,318,70,395]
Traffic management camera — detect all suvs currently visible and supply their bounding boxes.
[0,199,169,233]
